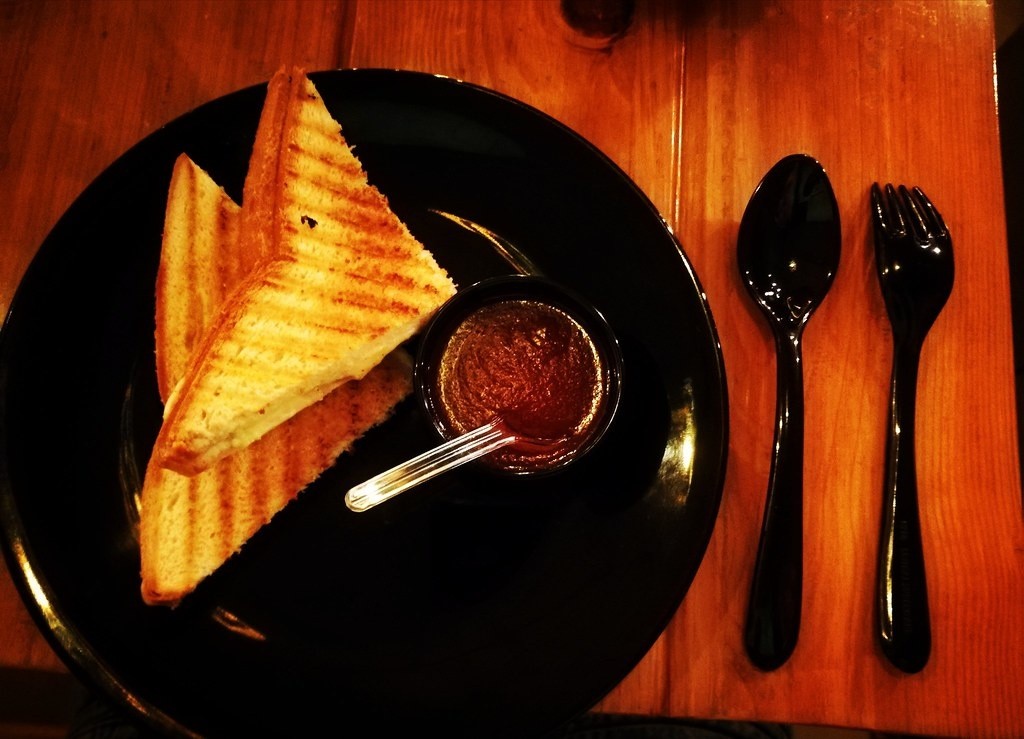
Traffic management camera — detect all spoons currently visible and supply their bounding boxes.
[737,154,843,673]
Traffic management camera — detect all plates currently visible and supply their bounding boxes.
[0,66,730,737]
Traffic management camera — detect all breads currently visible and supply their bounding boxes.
[140,62,458,606]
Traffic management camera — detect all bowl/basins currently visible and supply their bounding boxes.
[415,272,625,480]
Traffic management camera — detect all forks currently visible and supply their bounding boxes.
[870,182,955,673]
